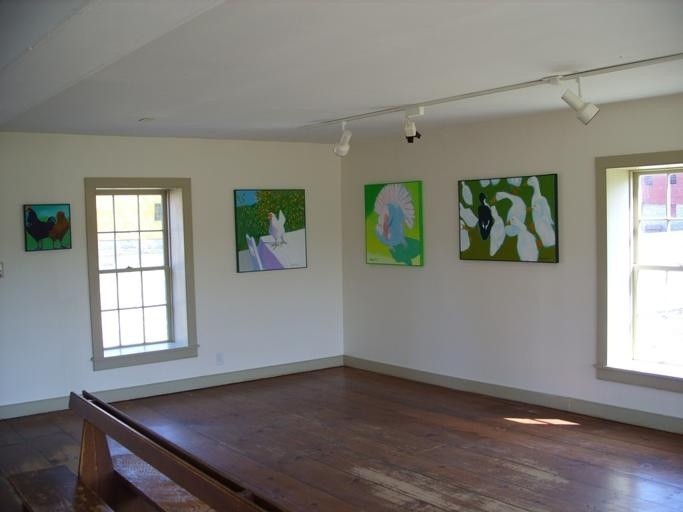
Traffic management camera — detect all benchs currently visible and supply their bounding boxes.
[5,388,287,512]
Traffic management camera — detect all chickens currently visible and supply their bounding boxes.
[267,210,287,248]
[24,205,69,251]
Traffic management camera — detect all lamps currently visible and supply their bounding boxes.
[560,78,600,128]
[403,108,426,143]
[333,120,352,159]
